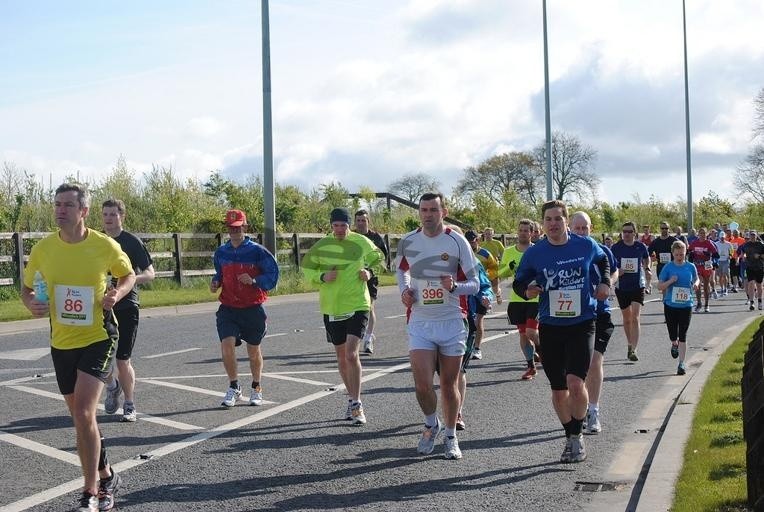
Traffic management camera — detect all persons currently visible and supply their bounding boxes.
[299,207,381,425]
[99,197,156,422]
[350,210,389,353]
[20,181,136,512]
[209,209,280,408]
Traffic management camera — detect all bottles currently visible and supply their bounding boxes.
[31,270,50,318]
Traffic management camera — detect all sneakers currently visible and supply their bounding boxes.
[453,412,468,433]
[693,284,763,312]
[248,388,263,407]
[442,435,463,461]
[98,464,122,511]
[585,407,605,437]
[352,400,367,424]
[520,364,539,384]
[469,344,483,360]
[415,415,442,456]
[344,396,354,420]
[73,487,100,512]
[121,400,137,423]
[104,376,124,415]
[220,385,244,408]
[624,342,690,377]
[560,431,587,467]
[361,331,379,354]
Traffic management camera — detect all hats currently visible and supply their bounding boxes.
[464,229,480,244]
[328,207,352,225]
[224,208,249,229]
[719,231,726,238]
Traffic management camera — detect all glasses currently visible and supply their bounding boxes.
[661,227,669,230]
[621,229,636,234]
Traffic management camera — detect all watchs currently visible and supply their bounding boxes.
[252,279,256,286]
